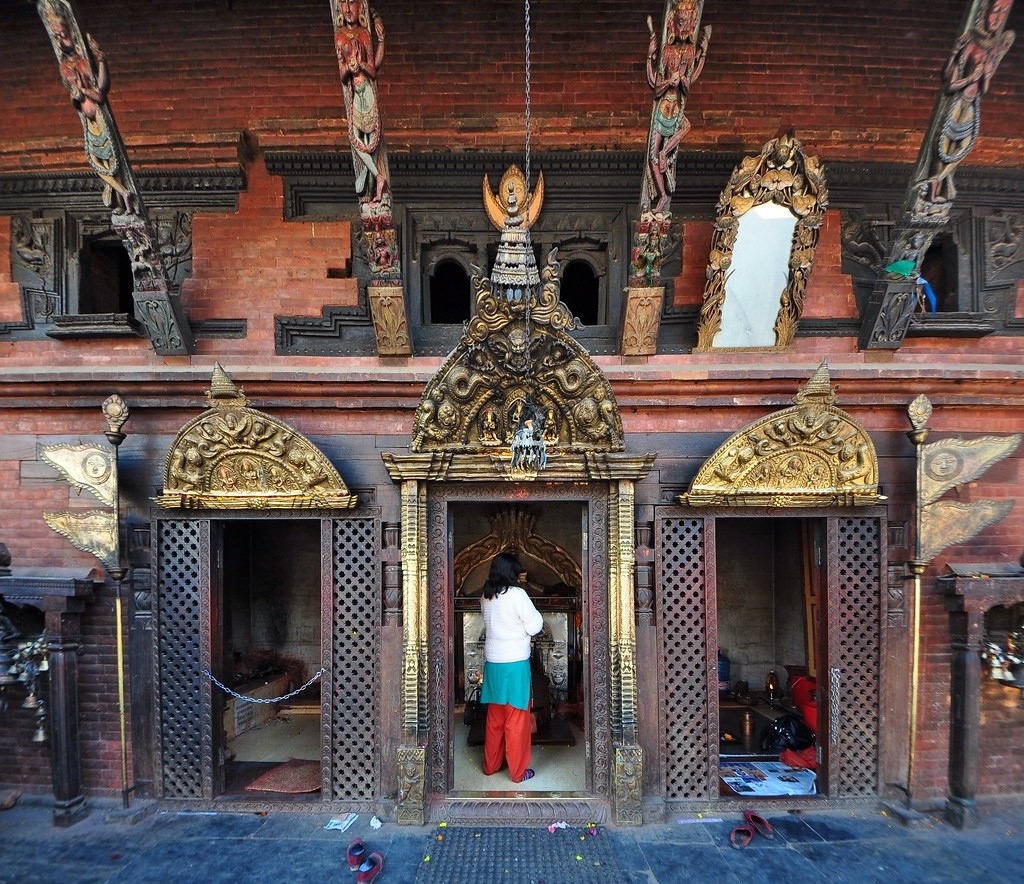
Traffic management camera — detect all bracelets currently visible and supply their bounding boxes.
[360,62,366,70]
[983,73,991,81]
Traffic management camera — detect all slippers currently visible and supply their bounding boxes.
[519,768,535,782]
[743,810,774,841]
[495,760,509,772]
[730,825,754,850]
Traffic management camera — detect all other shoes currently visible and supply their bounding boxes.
[357,851,385,884]
[347,837,366,871]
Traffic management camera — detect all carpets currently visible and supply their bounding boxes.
[245,759,322,794]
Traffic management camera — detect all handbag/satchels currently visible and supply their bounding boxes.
[466,710,486,747]
[463,686,483,727]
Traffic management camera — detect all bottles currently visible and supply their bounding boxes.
[765,671,777,700]
[717,648,730,694]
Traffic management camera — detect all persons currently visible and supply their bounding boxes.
[479,553,544,783]
[646,0,709,214]
[927,0,1016,204]
[333,0,386,202]
[42,0,133,215]
